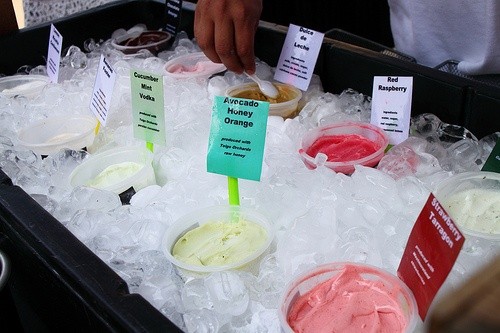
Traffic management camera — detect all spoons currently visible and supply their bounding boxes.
[243,70,280,99]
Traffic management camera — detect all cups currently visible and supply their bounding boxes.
[111,30,172,57]
[0,75,52,99]
[225,82,303,121]
[301,122,389,175]
[279,262,419,333]
[18,113,98,161]
[69,145,157,205]
[163,52,226,78]
[161,205,274,283]
[435,171,500,251]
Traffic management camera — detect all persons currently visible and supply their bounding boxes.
[194,0,500,75]
